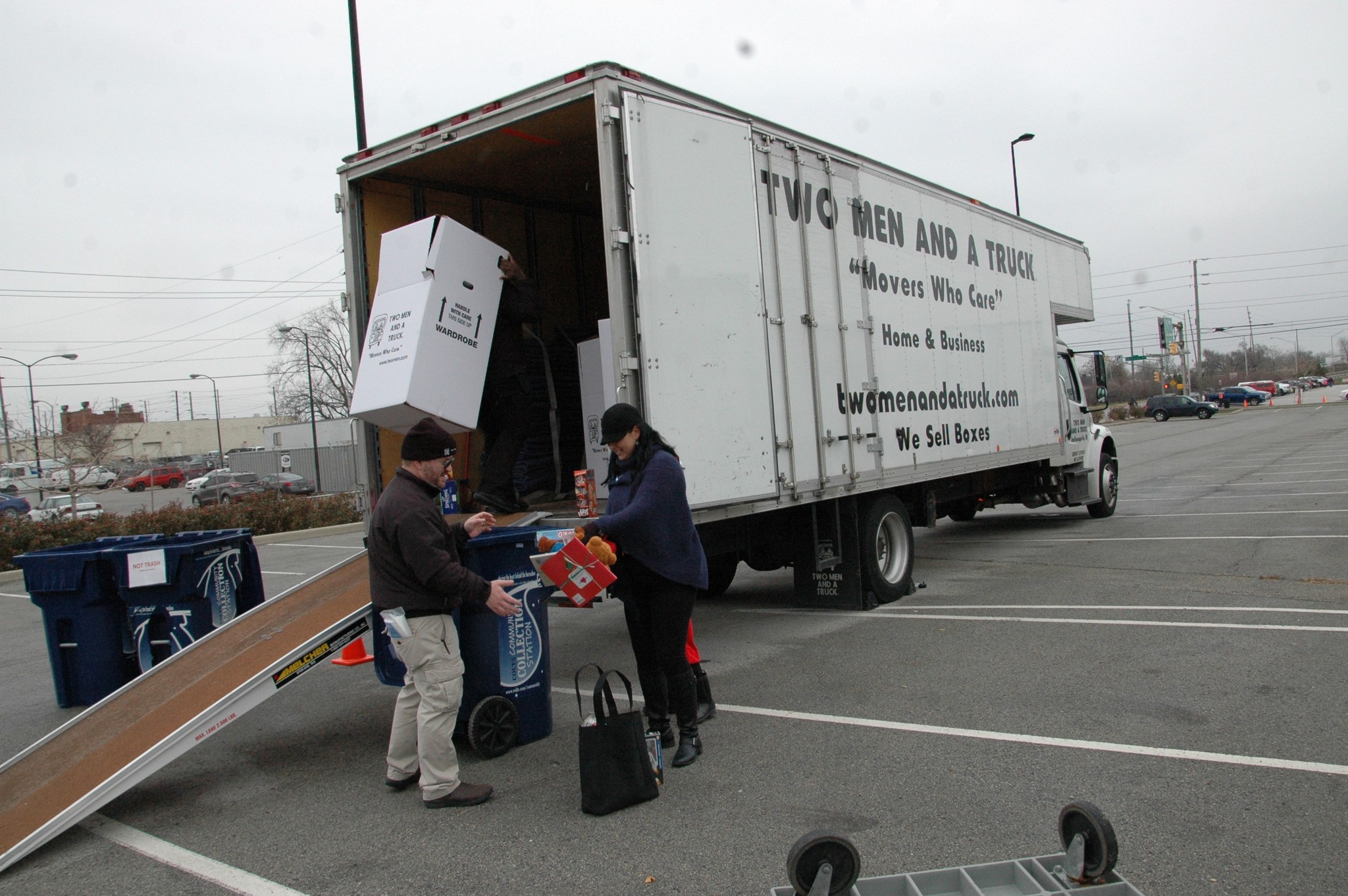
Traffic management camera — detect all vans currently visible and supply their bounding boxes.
[1237,381,1277,397]
[52,465,118,492]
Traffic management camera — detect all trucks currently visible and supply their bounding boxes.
[334,63,1120,614]
[0,458,69,496]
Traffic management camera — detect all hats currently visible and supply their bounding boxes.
[601,402,640,446]
[400,417,457,460]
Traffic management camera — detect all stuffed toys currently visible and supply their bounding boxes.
[538,526,617,566]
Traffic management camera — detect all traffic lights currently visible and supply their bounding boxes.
[1164,384,1169,389]
[1167,343,1176,355]
[1154,372,1160,382]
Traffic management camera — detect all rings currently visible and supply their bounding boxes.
[481,522,484,526]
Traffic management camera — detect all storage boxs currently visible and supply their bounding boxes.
[438,480,461,516]
[573,468,599,519]
[574,316,616,501]
[345,213,515,441]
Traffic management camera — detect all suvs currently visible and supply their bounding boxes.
[1274,376,1329,396]
[119,447,265,482]
[123,466,185,491]
[1144,396,1219,422]
[191,471,265,511]
[1208,386,1271,407]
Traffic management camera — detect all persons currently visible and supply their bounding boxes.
[601,537,717,724]
[1327,376,1333,387]
[1128,396,1137,407]
[367,417,522,809]
[581,403,709,767]
[472,254,541,511]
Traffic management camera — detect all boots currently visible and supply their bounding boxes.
[695,670,716,723]
[666,667,702,765]
[636,667,675,748]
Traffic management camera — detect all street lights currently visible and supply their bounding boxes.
[1139,306,1191,396]
[0,355,78,504]
[190,374,224,469]
[1269,337,1298,385]
[29,400,57,457]
[279,326,327,503]
[1011,133,1035,216]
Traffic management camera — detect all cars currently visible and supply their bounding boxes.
[259,473,315,497]
[27,495,104,526]
[1338,390,1348,399]
[0,493,31,522]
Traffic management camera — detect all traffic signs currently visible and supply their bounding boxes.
[1115,355,1122,364]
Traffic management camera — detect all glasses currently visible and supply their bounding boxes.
[434,458,455,469]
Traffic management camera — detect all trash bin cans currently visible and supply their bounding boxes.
[362,526,558,758]
[13,530,266,710]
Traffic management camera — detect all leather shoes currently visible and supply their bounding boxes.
[423,782,492,809]
[385,766,421,790]
[514,499,528,512]
[473,492,520,513]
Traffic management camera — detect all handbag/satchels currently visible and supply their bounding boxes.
[575,663,659,816]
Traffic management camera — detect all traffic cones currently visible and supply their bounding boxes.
[330,636,373,666]
[1243,400,1247,407]
[1297,397,1300,404]
[1323,396,1327,403]
[1269,398,1273,407]
